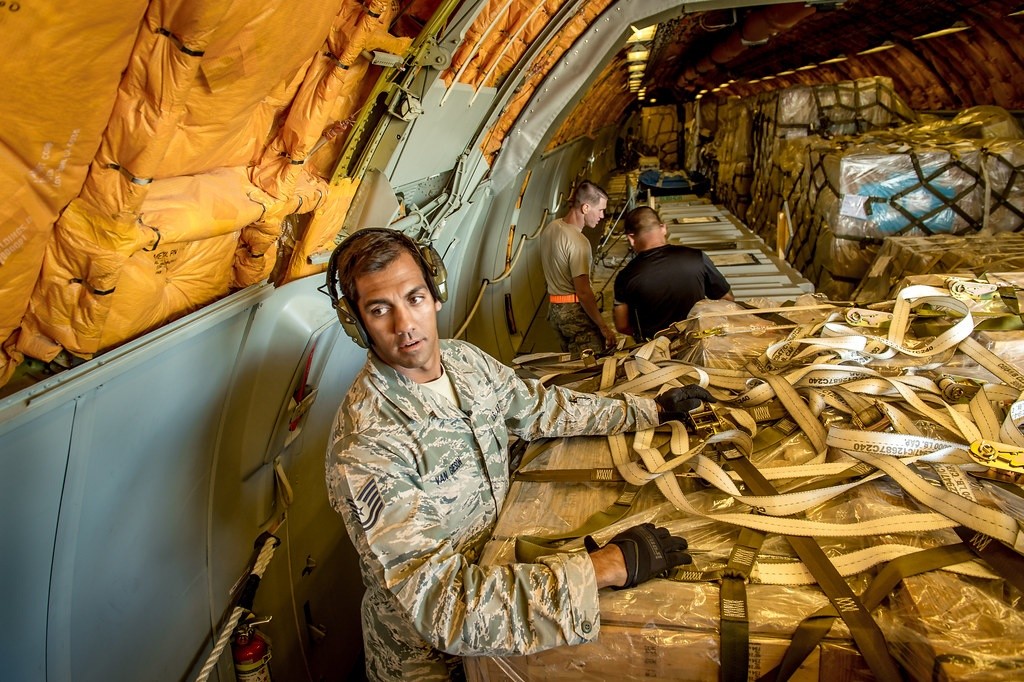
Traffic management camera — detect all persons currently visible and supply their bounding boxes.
[613,205,733,342]
[536,181,617,355]
[324,226,716,682]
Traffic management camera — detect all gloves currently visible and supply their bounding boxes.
[584,523,692,590]
[654,385,717,424]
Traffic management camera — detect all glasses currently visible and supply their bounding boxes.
[628,233,634,252]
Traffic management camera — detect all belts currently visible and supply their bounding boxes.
[549,294,579,304]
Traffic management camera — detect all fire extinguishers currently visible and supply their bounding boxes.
[231,616,276,682]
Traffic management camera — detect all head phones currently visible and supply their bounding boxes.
[324,227,448,349]
